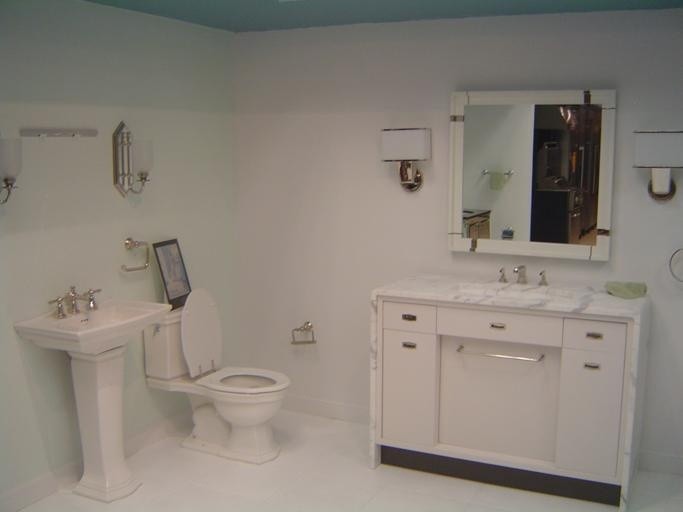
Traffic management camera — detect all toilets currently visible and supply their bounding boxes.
[145,290,290,464]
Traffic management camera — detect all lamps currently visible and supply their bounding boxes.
[0,138,24,204]
[630,130,682,201]
[111,119,155,200]
[378,126,432,195]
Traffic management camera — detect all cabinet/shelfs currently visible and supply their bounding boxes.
[377,301,439,452]
[436,306,563,463]
[558,315,627,480]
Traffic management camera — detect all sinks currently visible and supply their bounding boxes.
[51,306,148,330]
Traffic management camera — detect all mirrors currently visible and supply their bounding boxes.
[445,89,617,262]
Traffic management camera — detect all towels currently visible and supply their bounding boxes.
[488,168,505,191]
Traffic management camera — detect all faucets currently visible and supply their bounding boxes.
[69,285,95,313]
[513,264,528,283]
